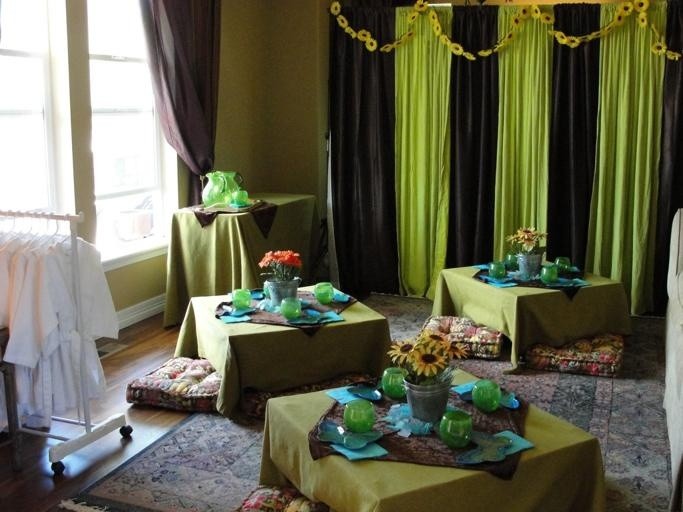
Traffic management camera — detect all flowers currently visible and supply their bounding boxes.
[506,228,547,255]
[257,249,303,282]
[386,330,470,386]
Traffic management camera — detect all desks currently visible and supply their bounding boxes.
[163,193,319,328]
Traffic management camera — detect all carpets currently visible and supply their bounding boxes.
[57,293,672,512]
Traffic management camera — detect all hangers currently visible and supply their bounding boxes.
[0,210,74,253]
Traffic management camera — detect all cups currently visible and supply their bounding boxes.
[232,282,334,320]
[489,253,571,285]
[234,191,248,206]
[344,367,501,449]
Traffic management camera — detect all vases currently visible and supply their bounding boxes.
[404,379,451,423]
[516,255,543,277]
[265,279,300,307]
